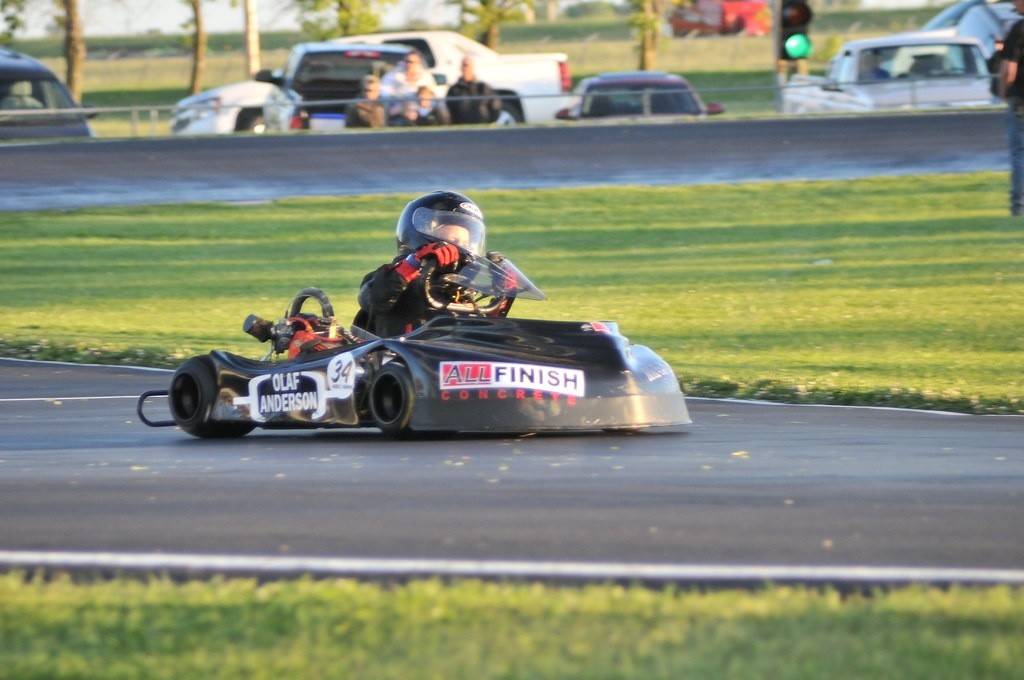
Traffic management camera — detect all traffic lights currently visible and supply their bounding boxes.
[772,0,814,61]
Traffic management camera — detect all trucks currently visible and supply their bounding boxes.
[779,33,999,113]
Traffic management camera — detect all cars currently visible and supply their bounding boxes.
[256,42,446,131]
[0,48,98,140]
[667,0,772,38]
[919,0,1023,57]
[171,31,575,136]
[556,72,725,119]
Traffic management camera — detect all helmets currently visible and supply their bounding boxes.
[396,190,486,275]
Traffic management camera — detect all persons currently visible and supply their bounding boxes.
[446,55,501,124]
[988,37,1004,97]
[989,0,1024,216]
[345,74,385,128]
[378,49,443,127]
[350,190,518,342]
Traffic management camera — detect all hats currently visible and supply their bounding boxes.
[362,74,381,90]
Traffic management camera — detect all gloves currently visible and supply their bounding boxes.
[394,241,459,283]
[491,267,518,316]
